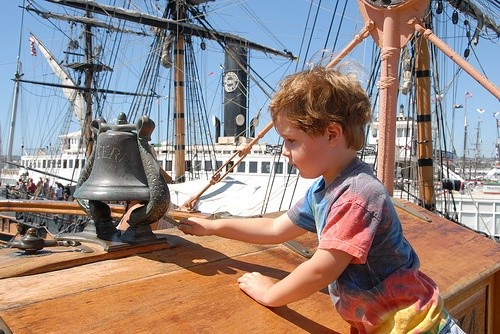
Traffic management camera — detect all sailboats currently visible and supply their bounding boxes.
[0,0,467,217]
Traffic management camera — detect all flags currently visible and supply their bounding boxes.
[477,108,485,113]
[493,112,499,117]
[465,91,472,97]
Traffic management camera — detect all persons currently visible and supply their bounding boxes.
[13,177,71,202]
[176,48,471,334]
